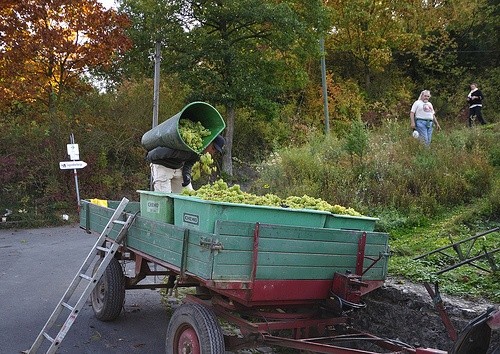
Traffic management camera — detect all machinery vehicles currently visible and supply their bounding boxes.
[76,199,500,354]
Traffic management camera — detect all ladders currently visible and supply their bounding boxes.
[26,198,134,354]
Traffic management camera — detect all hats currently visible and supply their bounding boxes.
[413,128,419,139]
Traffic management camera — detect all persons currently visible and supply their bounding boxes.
[410,90,441,148]
[468,82,488,128]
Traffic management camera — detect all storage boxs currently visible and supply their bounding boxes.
[167,194,331,236]
[325,214,380,232]
[136,190,180,225]
